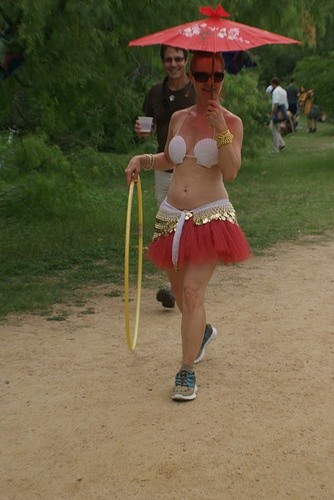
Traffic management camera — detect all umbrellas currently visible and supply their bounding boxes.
[129,3,303,112]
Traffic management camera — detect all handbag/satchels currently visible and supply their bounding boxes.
[272,103,287,124]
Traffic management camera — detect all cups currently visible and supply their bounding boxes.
[138,117,153,132]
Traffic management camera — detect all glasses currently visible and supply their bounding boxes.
[164,56,185,62]
[192,71,224,82]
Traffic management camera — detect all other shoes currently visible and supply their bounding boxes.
[156,288,175,308]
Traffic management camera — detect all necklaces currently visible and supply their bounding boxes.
[168,78,188,101]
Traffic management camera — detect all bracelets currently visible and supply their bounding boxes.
[216,129,233,147]
[145,153,153,170]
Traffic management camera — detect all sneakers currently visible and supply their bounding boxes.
[194,323,217,364]
[172,365,198,401]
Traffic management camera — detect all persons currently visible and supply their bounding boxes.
[136,45,197,308]
[284,78,317,133]
[266,78,289,153]
[126,52,251,400]
